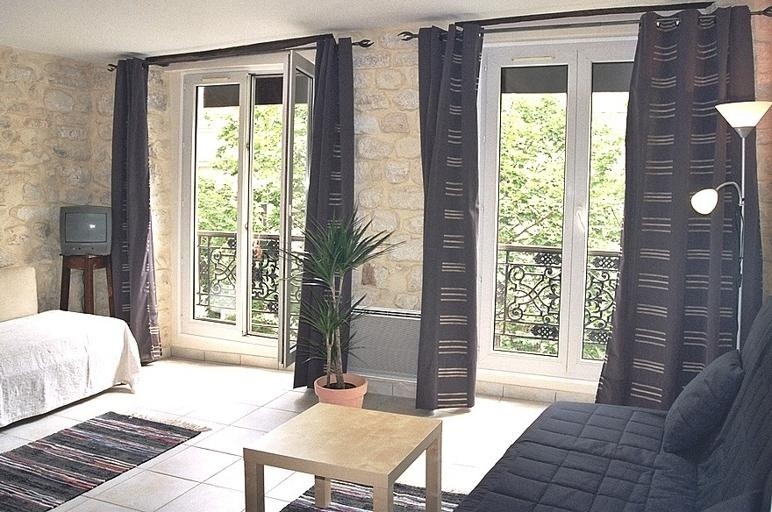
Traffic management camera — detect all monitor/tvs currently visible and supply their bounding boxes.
[60,206,112,256]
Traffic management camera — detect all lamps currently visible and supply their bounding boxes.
[690,97,772,354]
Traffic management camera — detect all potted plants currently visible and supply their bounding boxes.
[278,203,408,405]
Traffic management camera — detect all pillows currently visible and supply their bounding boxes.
[662,348,745,458]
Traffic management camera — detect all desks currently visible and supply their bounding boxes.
[59,255,119,319]
[240,404,445,511]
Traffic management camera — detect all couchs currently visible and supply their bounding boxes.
[0,264,135,432]
[452,299,770,511]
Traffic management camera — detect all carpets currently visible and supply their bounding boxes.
[276,475,469,508]
[0,406,211,512]
